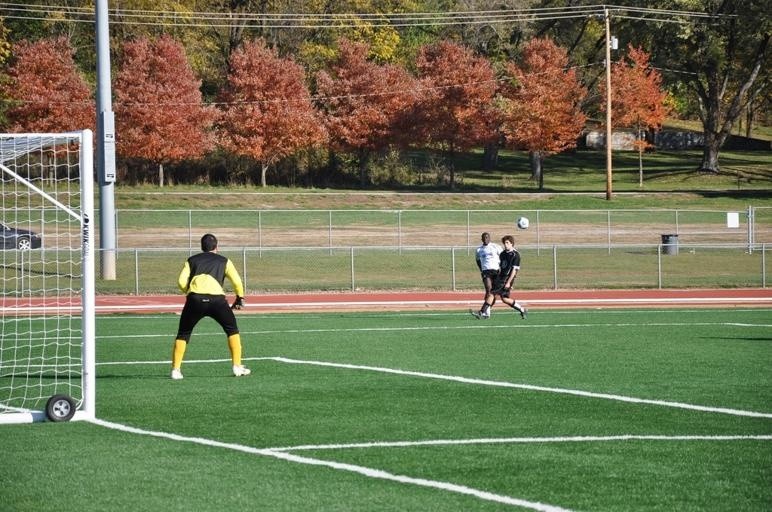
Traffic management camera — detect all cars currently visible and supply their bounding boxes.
[0,223,41,250]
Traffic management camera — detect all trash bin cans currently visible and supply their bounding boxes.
[662,235,678,254]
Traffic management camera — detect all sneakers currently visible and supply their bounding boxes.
[171,369,183,379]
[471,310,490,320]
[232,364,251,376]
[521,307,529,320]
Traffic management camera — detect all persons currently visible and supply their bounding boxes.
[171,234,250,380]
[471,236,528,320]
[476,232,503,318]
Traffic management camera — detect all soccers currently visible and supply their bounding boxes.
[518,217,529,230]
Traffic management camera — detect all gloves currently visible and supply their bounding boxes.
[231,295,245,311]
